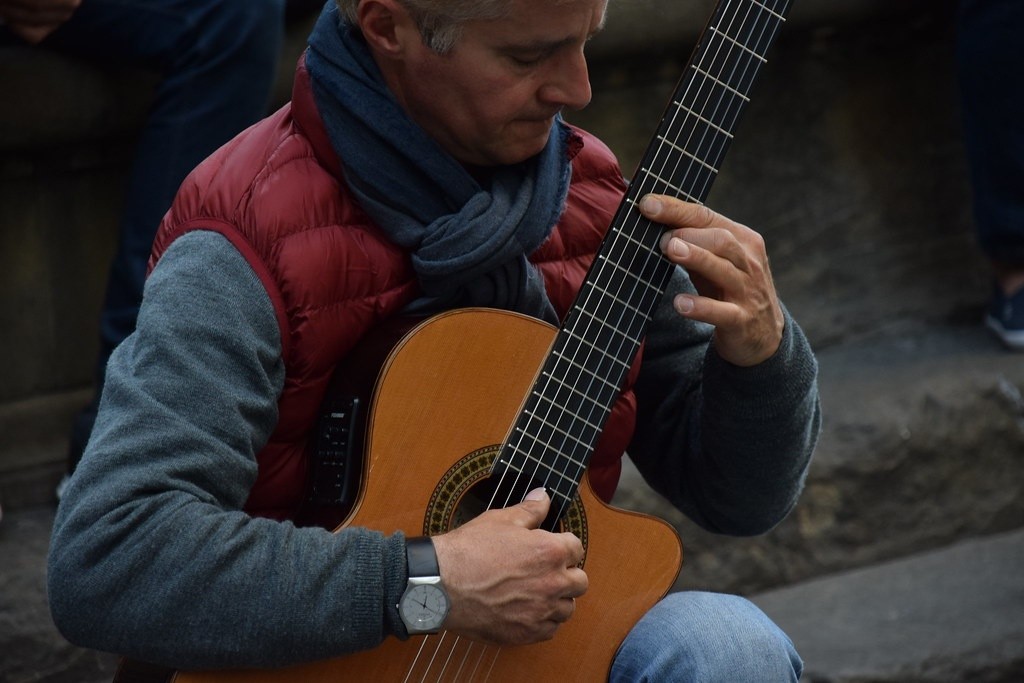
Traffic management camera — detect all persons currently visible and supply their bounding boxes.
[47,0,822,683]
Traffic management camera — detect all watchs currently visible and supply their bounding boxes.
[394,536,452,638]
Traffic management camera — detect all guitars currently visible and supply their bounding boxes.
[169,0,788,683]
